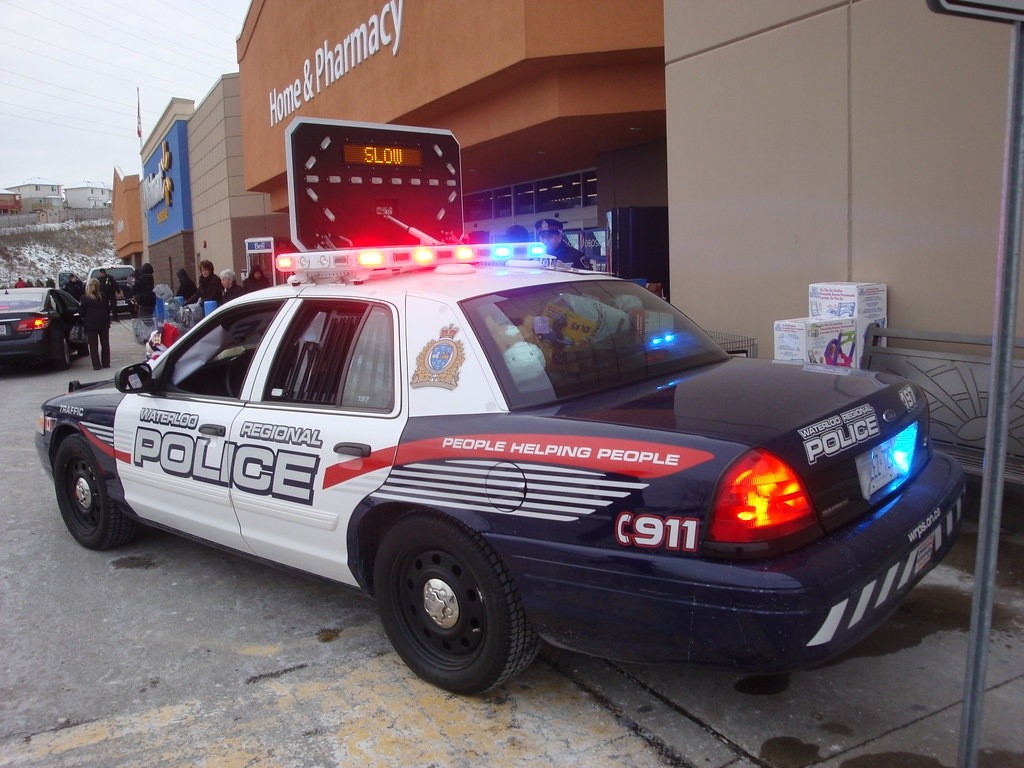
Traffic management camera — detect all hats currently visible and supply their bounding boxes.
[535,219,563,236]
[100,269,105,273]
[69,274,74,277]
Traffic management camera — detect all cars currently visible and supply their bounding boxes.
[34,235,969,694]
[0,287,89,371]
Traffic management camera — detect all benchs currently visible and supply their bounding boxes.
[861,323,1024,486]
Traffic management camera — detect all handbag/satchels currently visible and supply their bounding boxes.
[70,311,88,343]
[115,290,124,300]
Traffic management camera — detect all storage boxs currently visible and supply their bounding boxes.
[808,282,887,368]
[773,317,856,368]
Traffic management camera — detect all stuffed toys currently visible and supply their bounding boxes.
[485,315,555,384]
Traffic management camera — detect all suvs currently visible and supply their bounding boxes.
[55,264,139,317]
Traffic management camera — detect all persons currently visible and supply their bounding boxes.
[46,277,55,287]
[25,278,34,287]
[183,260,223,317]
[96,268,122,323]
[131,263,156,326]
[506,225,529,254]
[242,266,270,294]
[221,269,243,306]
[174,270,197,302]
[77,278,112,370]
[62,272,84,302]
[14,277,26,288]
[35,277,44,287]
[534,218,594,272]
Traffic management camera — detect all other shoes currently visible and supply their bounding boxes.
[94,365,110,370]
